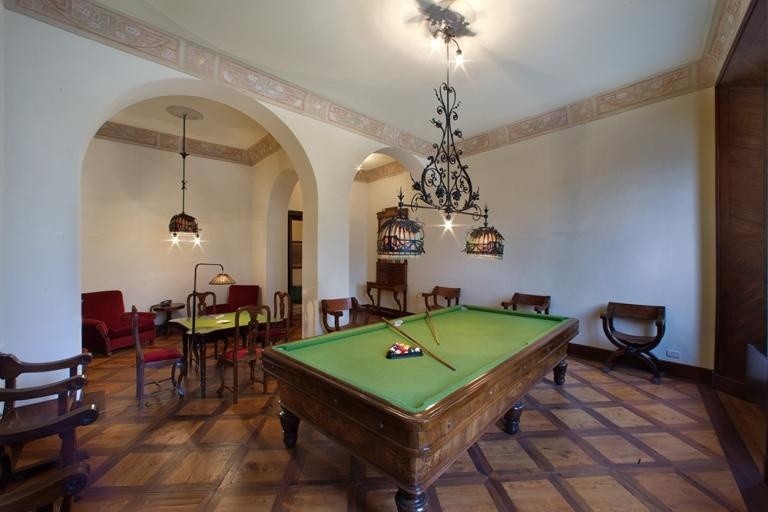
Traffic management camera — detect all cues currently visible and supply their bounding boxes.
[382,316,457,371]
[426,307,441,344]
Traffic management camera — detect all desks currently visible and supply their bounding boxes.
[260,304,580,512]
[367,259,407,313]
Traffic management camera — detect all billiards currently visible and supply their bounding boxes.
[385,343,424,359]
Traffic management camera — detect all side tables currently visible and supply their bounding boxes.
[153,303,186,339]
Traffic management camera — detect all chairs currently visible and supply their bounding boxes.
[132,305,189,407]
[218,304,271,404]
[600,301,666,384]
[204,285,262,313]
[257,290,291,345]
[0,352,98,512]
[422,286,461,311]
[186,291,228,372]
[501,292,551,314]
[322,297,369,333]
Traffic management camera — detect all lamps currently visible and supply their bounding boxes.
[378,26,504,258]
[193,263,236,340]
[167,105,203,244]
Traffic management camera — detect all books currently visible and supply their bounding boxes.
[205,312,224,319]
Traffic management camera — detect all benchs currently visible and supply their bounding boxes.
[81,290,158,356]
